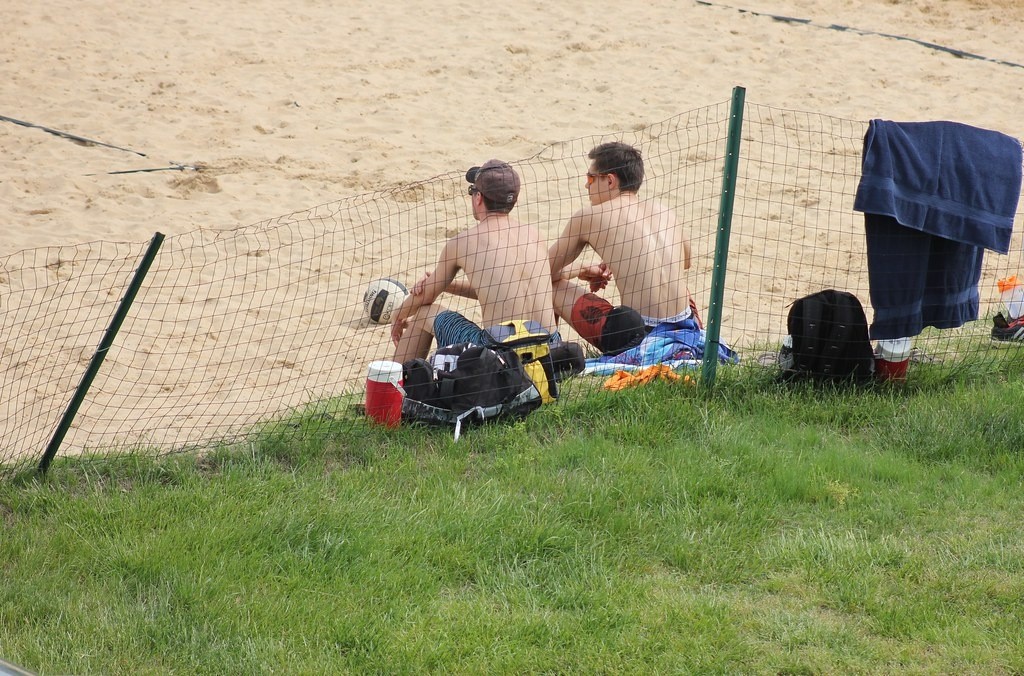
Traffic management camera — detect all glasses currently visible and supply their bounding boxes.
[468,185,478,195]
[587,173,617,183]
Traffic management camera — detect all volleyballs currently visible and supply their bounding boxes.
[363,277,411,324]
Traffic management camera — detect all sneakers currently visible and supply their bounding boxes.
[990,312,1024,349]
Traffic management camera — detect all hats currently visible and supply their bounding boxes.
[466,159,520,204]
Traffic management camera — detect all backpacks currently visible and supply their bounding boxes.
[401,342,542,443]
[770,290,878,395]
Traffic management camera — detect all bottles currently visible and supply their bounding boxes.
[997,276,1024,321]
[874,337,911,381]
[367,361,408,426]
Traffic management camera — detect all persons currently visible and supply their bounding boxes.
[548,142,703,356]
[391,159,556,369]
